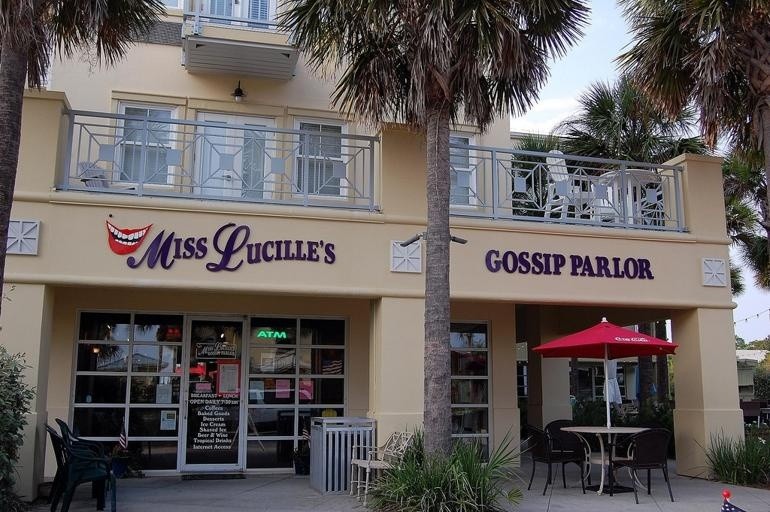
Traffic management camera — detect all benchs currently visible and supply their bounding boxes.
[350,431,423,508]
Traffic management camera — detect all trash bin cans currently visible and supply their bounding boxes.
[310,417,376,496]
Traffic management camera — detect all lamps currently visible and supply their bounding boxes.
[230,80,247,103]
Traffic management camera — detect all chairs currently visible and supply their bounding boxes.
[544,150,594,224]
[545,420,592,489]
[590,184,620,224]
[525,424,586,495]
[76,161,136,190]
[608,428,674,504]
[38,418,117,512]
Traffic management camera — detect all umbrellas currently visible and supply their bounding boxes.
[532,317,679,444]
